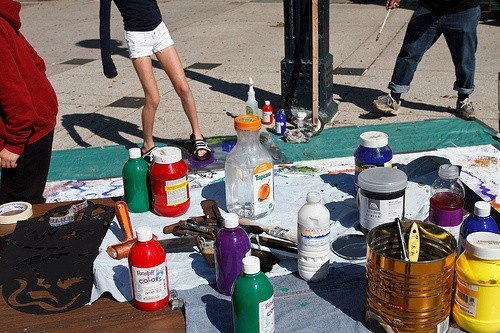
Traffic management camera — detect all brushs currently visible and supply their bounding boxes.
[106,200,158,260]
[239,217,298,244]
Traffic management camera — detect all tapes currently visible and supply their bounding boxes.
[0,201,33,224]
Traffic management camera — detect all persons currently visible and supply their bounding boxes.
[372,0,483,118]
[113,0,213,162]
[0,0,59,204]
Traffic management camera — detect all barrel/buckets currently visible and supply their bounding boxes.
[365,219,457,333]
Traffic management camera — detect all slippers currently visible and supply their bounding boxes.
[140,146,159,163]
[190,133,211,161]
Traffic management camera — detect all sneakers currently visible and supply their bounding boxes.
[456,98,476,120]
[373,93,401,115]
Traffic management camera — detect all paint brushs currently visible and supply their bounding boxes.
[376,9,391,41]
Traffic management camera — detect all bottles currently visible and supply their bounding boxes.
[354,131,394,192]
[122,147,151,214]
[297,190,332,282]
[428,164,500,254]
[149,146,191,218]
[214,213,251,294]
[224,115,274,219]
[245,77,286,135]
[452,232,500,333]
[232,255,275,332]
[128,226,171,310]
